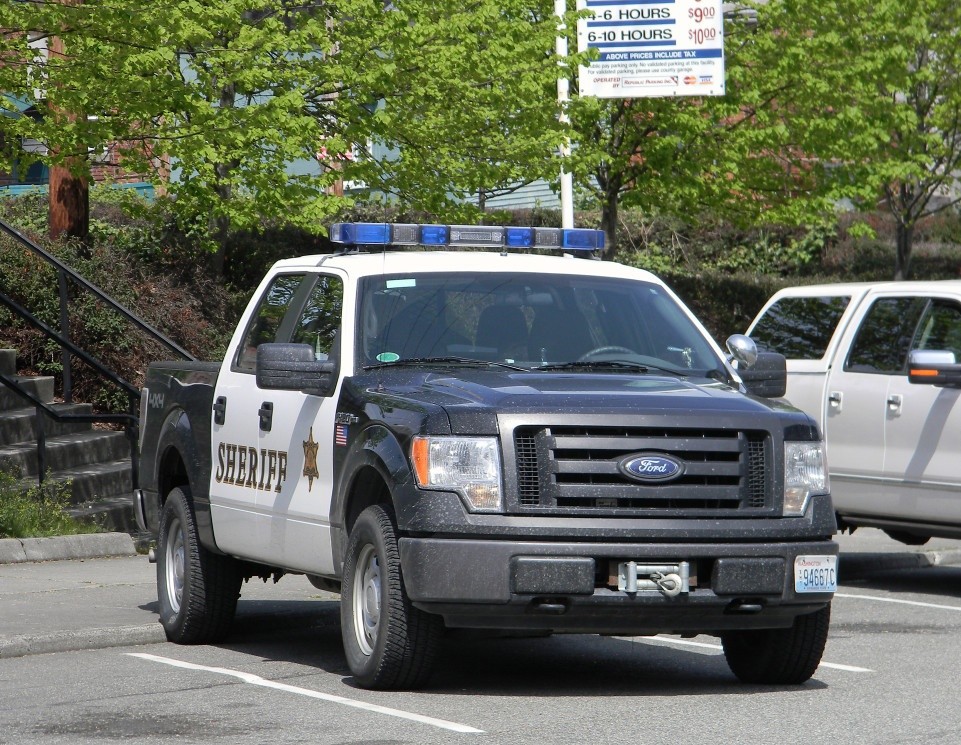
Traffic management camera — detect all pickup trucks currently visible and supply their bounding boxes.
[131,223,838,690]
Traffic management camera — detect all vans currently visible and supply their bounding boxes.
[727,280,960,540]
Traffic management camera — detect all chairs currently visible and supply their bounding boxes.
[474,301,528,348]
[386,319,447,359]
[527,308,592,364]
